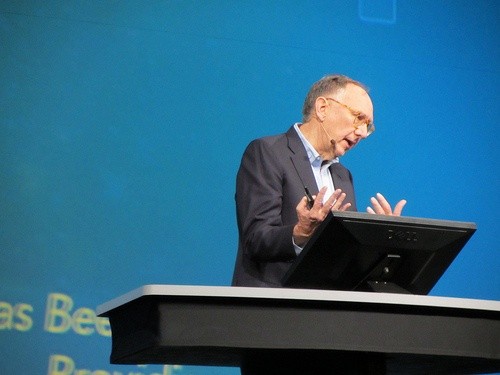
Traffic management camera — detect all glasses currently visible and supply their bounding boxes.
[326,96,376,138]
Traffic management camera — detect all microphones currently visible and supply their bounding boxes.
[319,121,336,145]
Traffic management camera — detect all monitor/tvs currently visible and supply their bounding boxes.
[280,210,476,295]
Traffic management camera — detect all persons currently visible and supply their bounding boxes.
[230,71,407,293]
